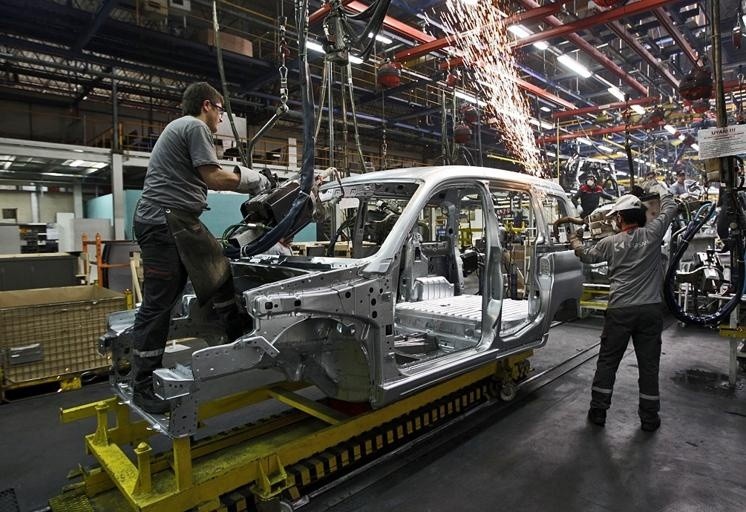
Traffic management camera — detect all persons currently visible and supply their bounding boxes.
[569,179,681,431]
[570,171,695,236]
[129,78,272,417]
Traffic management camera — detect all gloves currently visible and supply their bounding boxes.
[648,180,674,201]
[233,165,272,194]
[567,227,584,250]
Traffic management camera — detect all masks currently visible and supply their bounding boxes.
[610,218,622,232]
[587,181,593,186]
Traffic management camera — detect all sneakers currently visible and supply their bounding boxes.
[213,302,252,342]
[637,410,661,432]
[587,407,607,427]
[132,376,170,414]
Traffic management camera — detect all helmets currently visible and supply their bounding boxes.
[585,174,597,183]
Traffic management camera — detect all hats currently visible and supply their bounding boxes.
[605,194,642,218]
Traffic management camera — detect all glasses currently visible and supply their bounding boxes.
[211,102,226,112]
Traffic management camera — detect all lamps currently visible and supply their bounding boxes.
[597,87,699,181]
[450,0,594,180]
[368,32,393,45]
[297,38,364,65]
